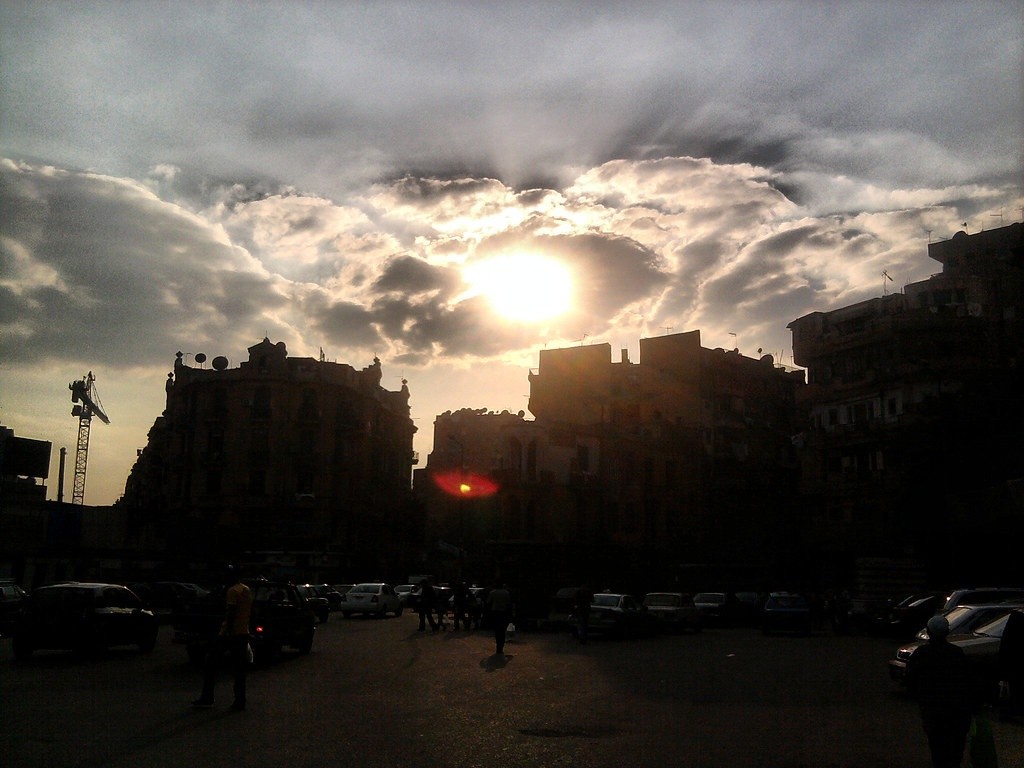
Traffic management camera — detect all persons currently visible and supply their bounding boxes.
[575,582,595,645]
[191,564,253,709]
[418,578,511,654]
[905,615,980,768]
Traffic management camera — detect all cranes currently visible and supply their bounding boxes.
[69,371,109,505]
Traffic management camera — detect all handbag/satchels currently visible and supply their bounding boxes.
[968,705,998,768]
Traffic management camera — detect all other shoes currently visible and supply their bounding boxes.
[191,696,215,706]
[231,697,246,710]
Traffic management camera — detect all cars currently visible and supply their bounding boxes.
[888,609,1024,709]
[341,583,404,618]
[762,591,810,633]
[640,592,692,631]
[876,587,1024,643]
[160,581,491,658]
[693,592,743,626]
[0,580,26,599]
[13,584,159,666]
[592,593,638,632]
[914,602,1024,644]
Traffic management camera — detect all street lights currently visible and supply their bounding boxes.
[446,434,464,579]
